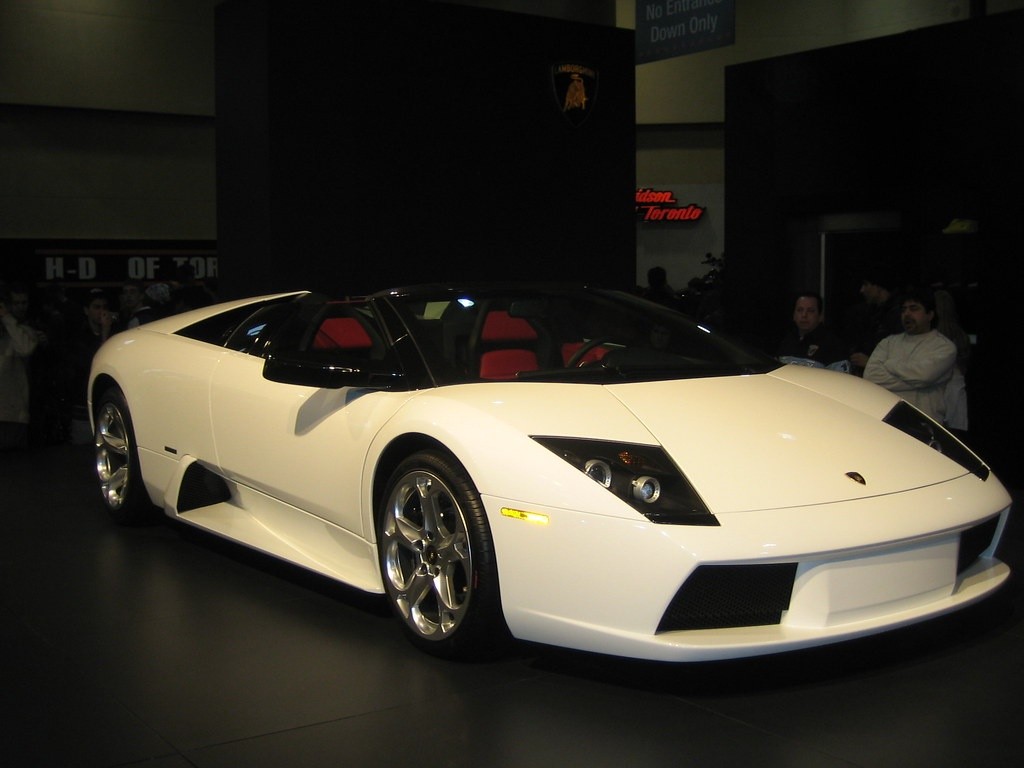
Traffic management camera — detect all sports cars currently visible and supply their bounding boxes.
[88,281,1014,668]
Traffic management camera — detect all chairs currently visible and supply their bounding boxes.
[460,298,553,383]
[298,303,390,370]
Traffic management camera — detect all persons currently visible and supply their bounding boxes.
[0,262,229,458]
[780,276,975,440]
[646,321,673,345]
[648,265,678,304]
